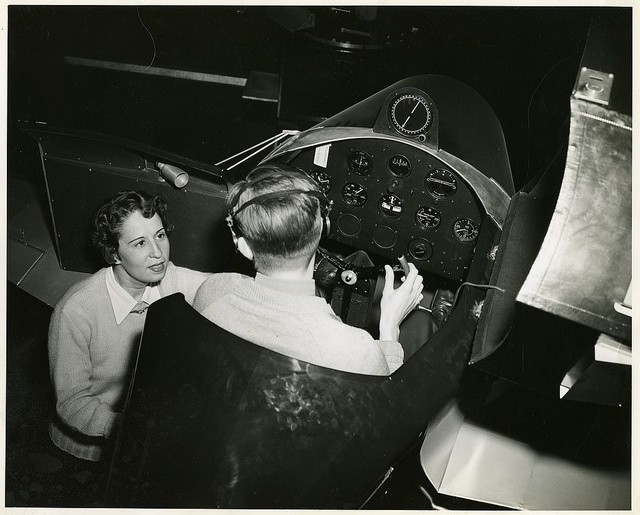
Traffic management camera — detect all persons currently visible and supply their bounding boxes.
[191,165,424,378]
[47,190,214,462]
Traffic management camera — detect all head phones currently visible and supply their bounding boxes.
[227,190,333,261]
